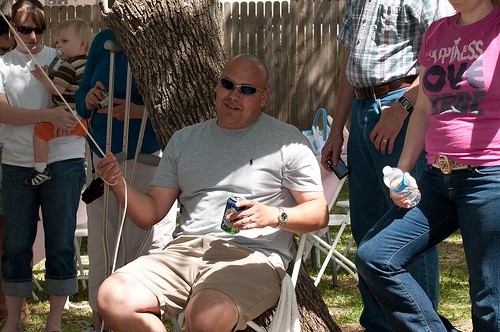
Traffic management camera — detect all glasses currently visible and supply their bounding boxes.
[15,25,43,34]
[218,76,264,95]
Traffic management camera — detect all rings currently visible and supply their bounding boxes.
[67,123,73,128]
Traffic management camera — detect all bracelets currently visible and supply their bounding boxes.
[37,109,42,123]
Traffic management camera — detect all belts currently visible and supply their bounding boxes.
[353,74,419,99]
[431,154,475,174]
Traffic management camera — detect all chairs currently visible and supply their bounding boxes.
[169,154,359,332]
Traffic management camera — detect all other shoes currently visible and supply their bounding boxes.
[24,168,51,185]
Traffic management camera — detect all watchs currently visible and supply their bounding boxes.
[272,205,289,230]
[398,96,414,114]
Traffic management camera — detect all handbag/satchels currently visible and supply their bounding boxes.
[301,108,329,153]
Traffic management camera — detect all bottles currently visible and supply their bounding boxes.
[383,165,421,208]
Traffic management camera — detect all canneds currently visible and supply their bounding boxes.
[221,195,247,234]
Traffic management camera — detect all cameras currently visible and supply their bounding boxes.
[327,156,351,180]
[97,92,110,109]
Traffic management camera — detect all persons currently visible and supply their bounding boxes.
[0,1,176,332]
[96,55,330,332]
[321,0,500,332]
